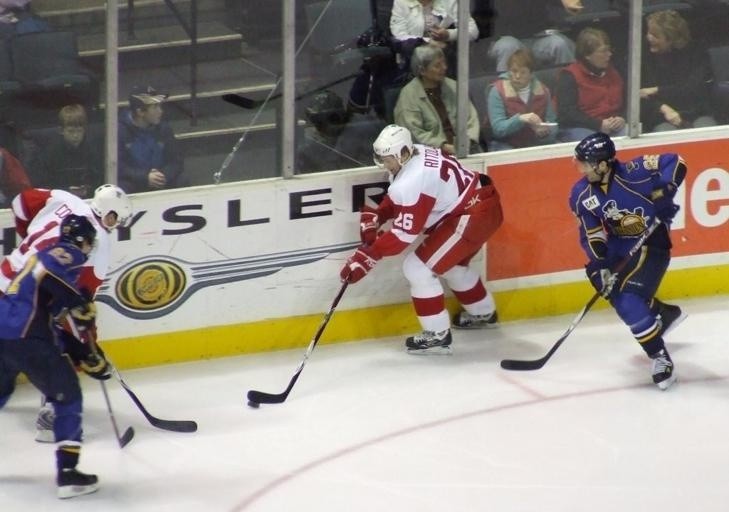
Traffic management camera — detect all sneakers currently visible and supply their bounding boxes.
[37,409,52,432]
[659,304,682,335]
[406,329,452,349]
[453,309,498,328]
[649,349,673,385]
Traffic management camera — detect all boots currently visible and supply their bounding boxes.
[54,448,98,486]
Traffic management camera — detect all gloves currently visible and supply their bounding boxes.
[584,261,614,300]
[359,204,384,245]
[79,350,109,383]
[340,248,379,283]
[649,183,680,218]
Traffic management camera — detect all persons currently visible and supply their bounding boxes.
[0,212,112,498]
[568,130,687,390]
[0,182,129,444]
[292,0,722,176]
[339,124,504,354]
[0,1,190,215]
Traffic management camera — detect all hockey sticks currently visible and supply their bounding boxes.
[87,333,134,449]
[246,279,348,402]
[95,350,197,432]
[500,215,662,370]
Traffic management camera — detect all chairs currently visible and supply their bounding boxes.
[0,1,192,209]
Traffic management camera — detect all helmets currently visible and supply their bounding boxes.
[92,184,134,233]
[59,214,96,251]
[574,133,616,171]
[373,124,415,167]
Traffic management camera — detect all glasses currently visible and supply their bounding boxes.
[593,47,613,54]
